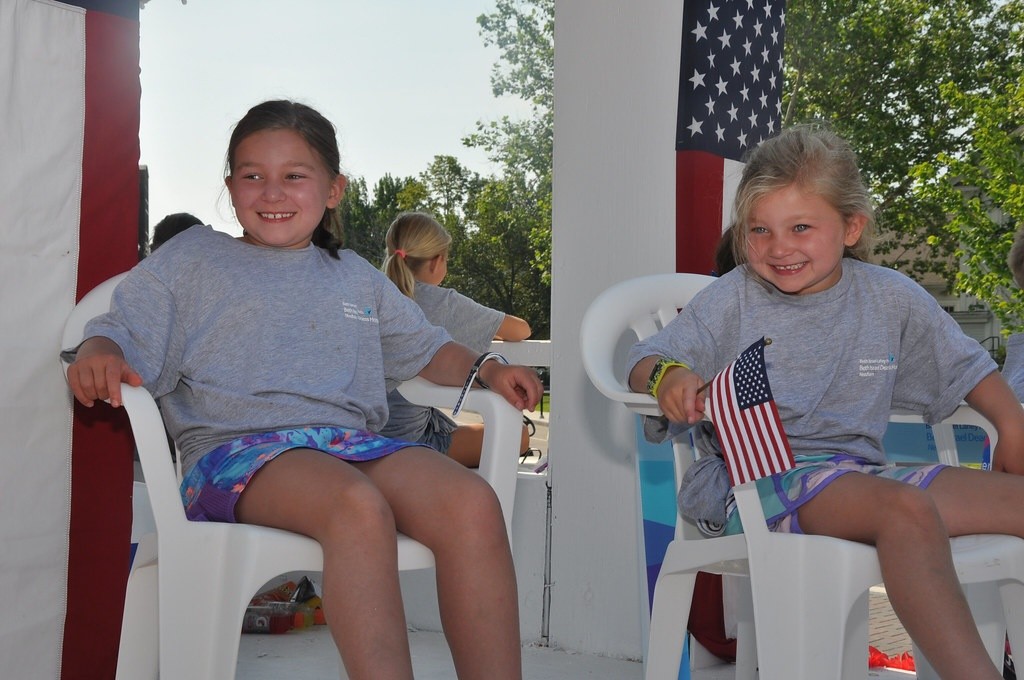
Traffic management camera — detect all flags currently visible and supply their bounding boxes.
[676,1,788,167]
[712,335,797,488]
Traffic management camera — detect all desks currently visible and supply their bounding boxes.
[458,325,551,378]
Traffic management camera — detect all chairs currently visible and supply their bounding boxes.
[577,270,1023,679]
[60,265,526,679]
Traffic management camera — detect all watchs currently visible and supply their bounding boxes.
[647,358,692,399]
[451,351,509,417]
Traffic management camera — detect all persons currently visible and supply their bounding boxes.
[51,99,547,680]
[614,119,1023,680]
[366,211,533,468]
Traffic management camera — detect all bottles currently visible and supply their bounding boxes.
[247,599,327,633]
[241,606,306,634]
